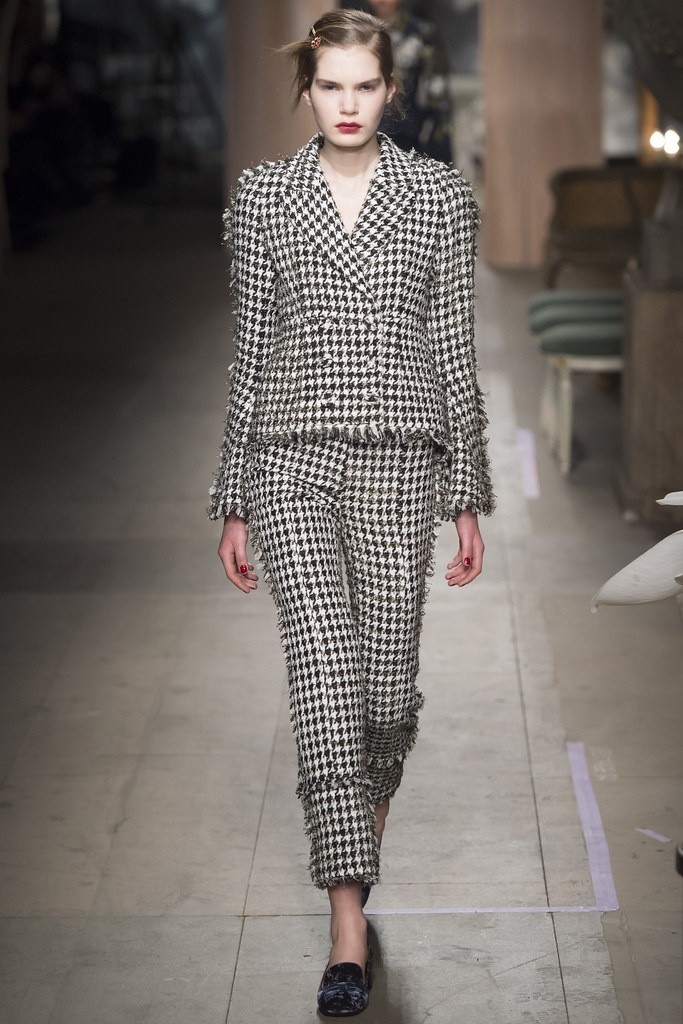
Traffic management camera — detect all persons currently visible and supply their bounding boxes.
[204,9,497,1017]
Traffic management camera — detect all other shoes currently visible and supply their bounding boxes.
[317,944,373,1016]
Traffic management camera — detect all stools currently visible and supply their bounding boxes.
[527,290,624,475]
